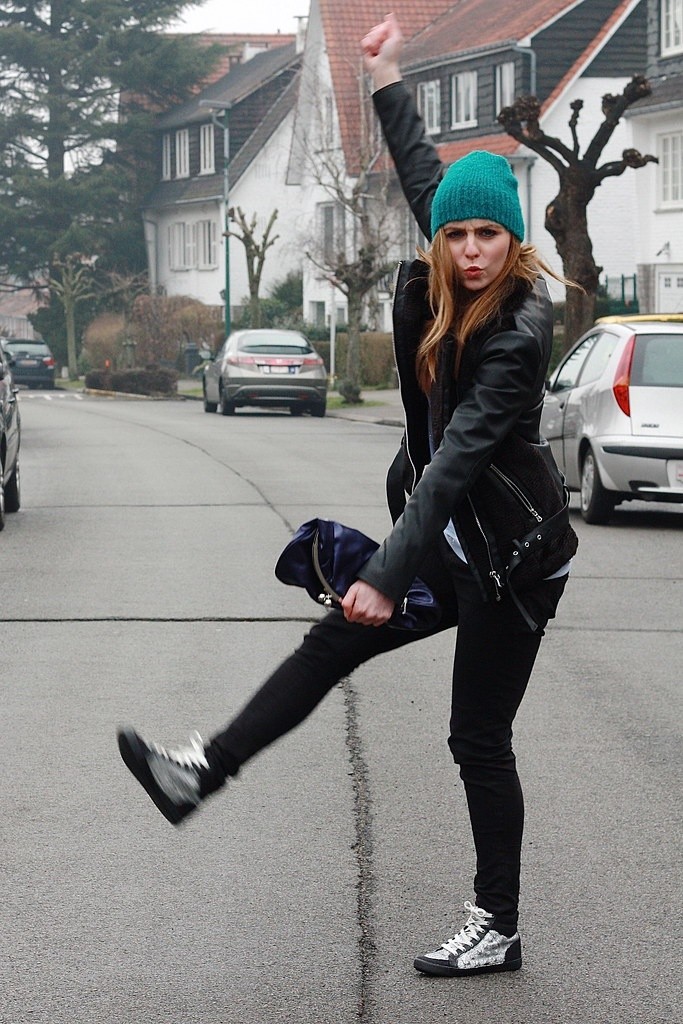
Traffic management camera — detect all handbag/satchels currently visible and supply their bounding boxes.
[275,516,438,634]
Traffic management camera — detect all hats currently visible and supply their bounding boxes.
[431,150,524,241]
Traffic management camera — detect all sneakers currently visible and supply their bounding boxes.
[412,900,524,977]
[119,725,227,827]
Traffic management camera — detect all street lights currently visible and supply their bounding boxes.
[198,98,233,341]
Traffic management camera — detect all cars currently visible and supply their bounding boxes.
[537,320,683,524]
[7,339,55,389]
[0,343,23,531]
[201,328,328,418]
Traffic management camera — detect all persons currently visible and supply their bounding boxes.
[119,13,579,979]
